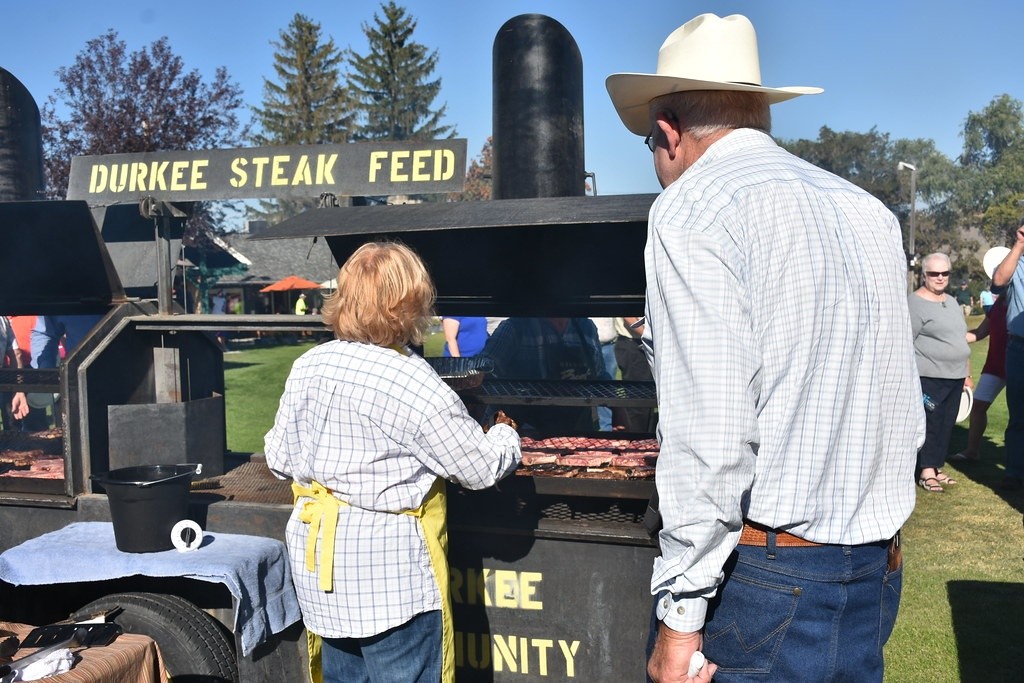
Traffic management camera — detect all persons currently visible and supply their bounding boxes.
[442,316,658,431]
[0,315,104,432]
[907,224,1024,491]
[263,241,523,683]
[211,289,318,346]
[605,12,926,683]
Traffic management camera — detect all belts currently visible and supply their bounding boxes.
[738,525,827,548]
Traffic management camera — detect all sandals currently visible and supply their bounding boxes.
[918,470,958,492]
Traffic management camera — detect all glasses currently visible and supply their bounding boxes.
[643,116,660,153]
[924,270,950,277]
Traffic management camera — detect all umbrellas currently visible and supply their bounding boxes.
[259,274,324,315]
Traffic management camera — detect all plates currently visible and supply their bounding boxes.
[955,386,974,423]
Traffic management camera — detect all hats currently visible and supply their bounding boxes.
[604,12,824,136]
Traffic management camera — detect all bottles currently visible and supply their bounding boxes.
[922,392,939,413]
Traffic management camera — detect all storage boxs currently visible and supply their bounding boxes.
[426,355,496,389]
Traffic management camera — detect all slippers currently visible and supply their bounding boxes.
[953,452,979,460]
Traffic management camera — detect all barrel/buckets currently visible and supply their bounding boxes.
[88,461,203,554]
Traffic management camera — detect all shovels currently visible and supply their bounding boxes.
[17,605,126,648]
